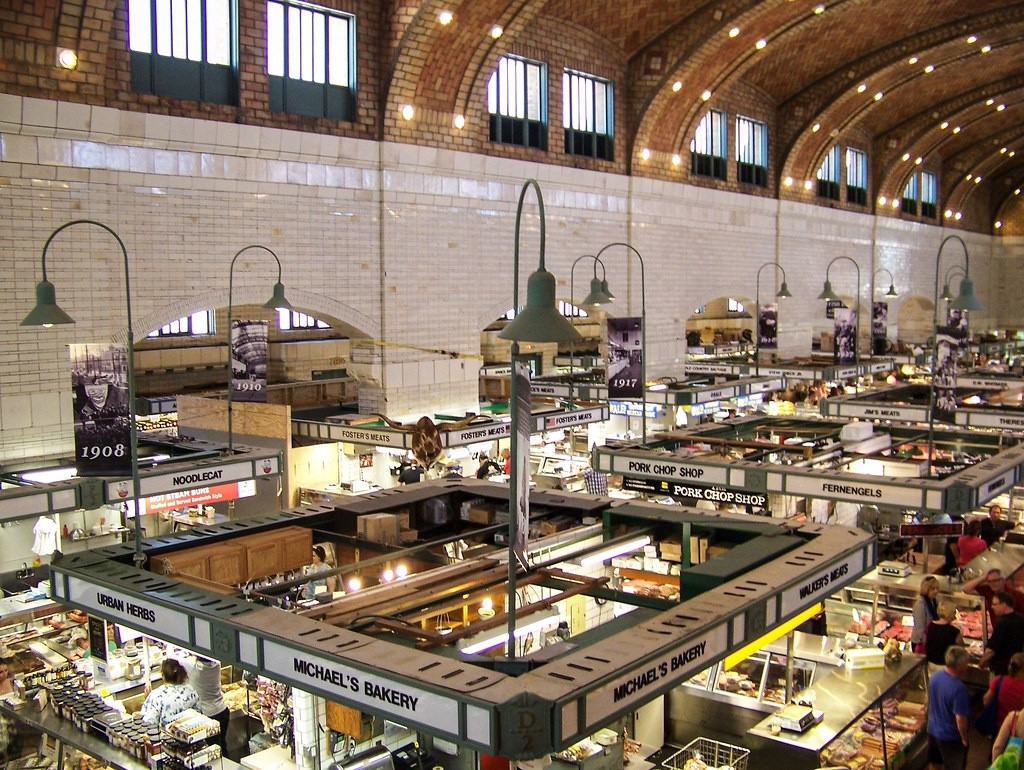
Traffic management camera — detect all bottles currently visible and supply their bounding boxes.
[23,663,77,691]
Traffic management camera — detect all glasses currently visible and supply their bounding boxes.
[991,602,1000,606]
[987,579,1000,584]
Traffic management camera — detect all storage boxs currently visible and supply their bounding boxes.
[0,361,1024,769]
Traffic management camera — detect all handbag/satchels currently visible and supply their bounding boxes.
[914,636,926,654]
[975,676,1003,741]
[986,711,1024,770]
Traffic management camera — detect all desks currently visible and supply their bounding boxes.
[197,375,354,405]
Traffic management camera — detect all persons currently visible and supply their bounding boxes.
[980,592,1024,682]
[475,448,511,478]
[69,628,118,657]
[169,651,230,760]
[926,599,965,679]
[925,646,972,770]
[304,546,337,600]
[924,504,1015,568]
[911,576,940,655]
[963,569,1024,631]
[140,659,201,726]
[783,380,846,406]
[577,457,609,496]
[983,651,1024,770]
[398,460,424,487]
[886,369,898,385]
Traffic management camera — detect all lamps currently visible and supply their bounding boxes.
[20,180,985,656]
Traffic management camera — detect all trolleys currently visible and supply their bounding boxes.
[661,736,750,770]
